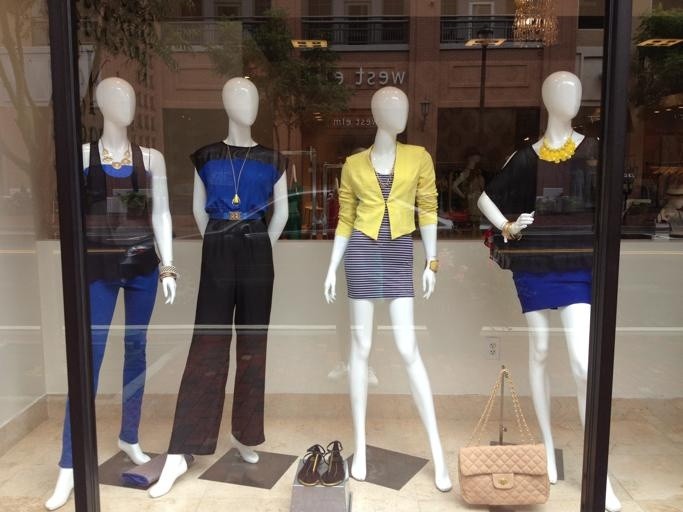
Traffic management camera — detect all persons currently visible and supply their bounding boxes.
[476,71,621,512]
[149,75,289,498]
[454,148,486,229]
[46,75,179,512]
[323,85,454,490]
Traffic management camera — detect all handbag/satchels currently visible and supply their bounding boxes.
[457,442,551,506]
[120,446,194,491]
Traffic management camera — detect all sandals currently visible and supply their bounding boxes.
[321,439,346,488]
[296,444,327,487]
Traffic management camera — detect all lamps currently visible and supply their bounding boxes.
[512,0,560,48]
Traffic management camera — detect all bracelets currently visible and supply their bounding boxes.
[422,259,440,272]
[503,221,522,242]
[158,272,177,281]
[157,266,178,273]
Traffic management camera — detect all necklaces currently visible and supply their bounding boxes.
[98,137,132,169]
[225,137,252,207]
[538,129,577,164]
[370,145,397,191]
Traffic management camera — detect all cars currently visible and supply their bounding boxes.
[106,188,456,298]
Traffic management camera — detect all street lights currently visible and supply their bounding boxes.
[473,25,491,175]
